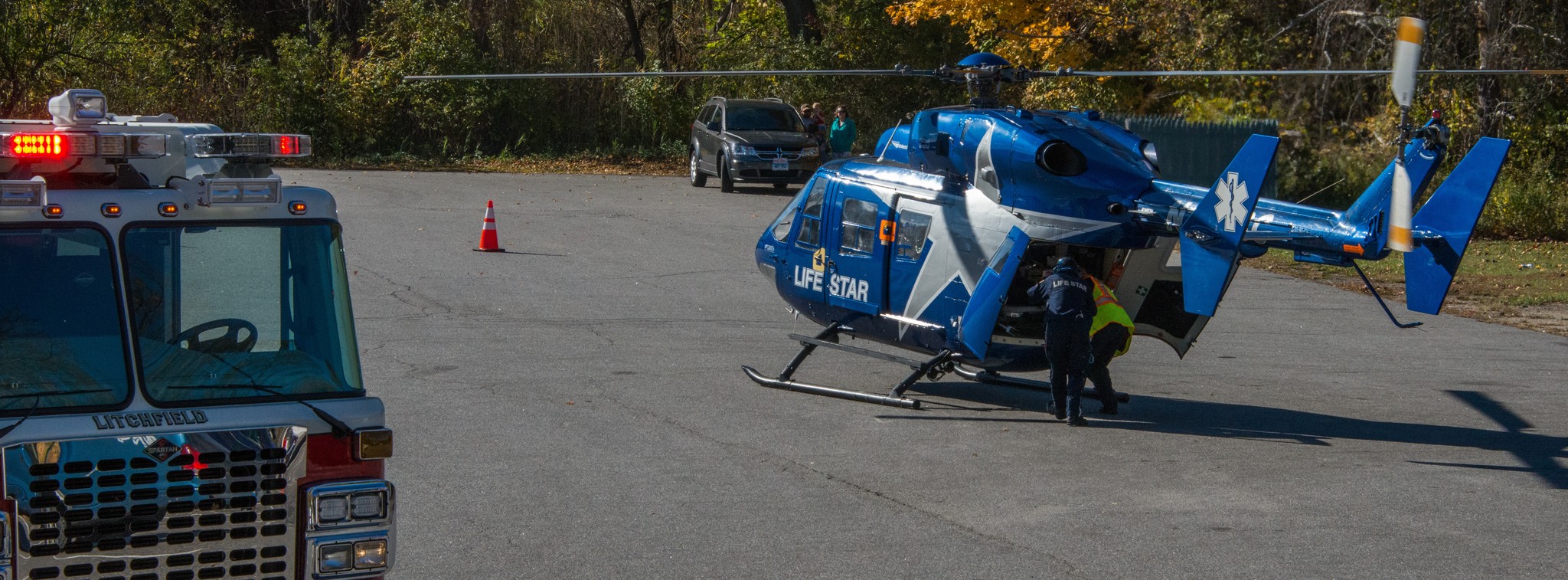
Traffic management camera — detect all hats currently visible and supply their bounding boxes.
[801,104,811,111]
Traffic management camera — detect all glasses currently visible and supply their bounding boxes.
[802,110,811,113]
[837,111,846,113]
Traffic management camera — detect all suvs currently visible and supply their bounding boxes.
[0,88,396,580]
[688,93,819,196]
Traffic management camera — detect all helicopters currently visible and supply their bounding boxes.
[402,53,1510,409]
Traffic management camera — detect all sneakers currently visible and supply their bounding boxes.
[1046,400,1067,420]
[1066,415,1084,427]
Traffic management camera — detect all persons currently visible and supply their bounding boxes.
[829,104,856,161]
[810,102,831,153]
[1073,265,1136,415]
[800,103,829,165]
[1026,256,1098,427]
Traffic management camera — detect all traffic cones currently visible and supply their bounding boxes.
[470,199,505,253]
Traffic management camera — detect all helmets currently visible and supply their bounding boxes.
[1052,256,1080,274]
